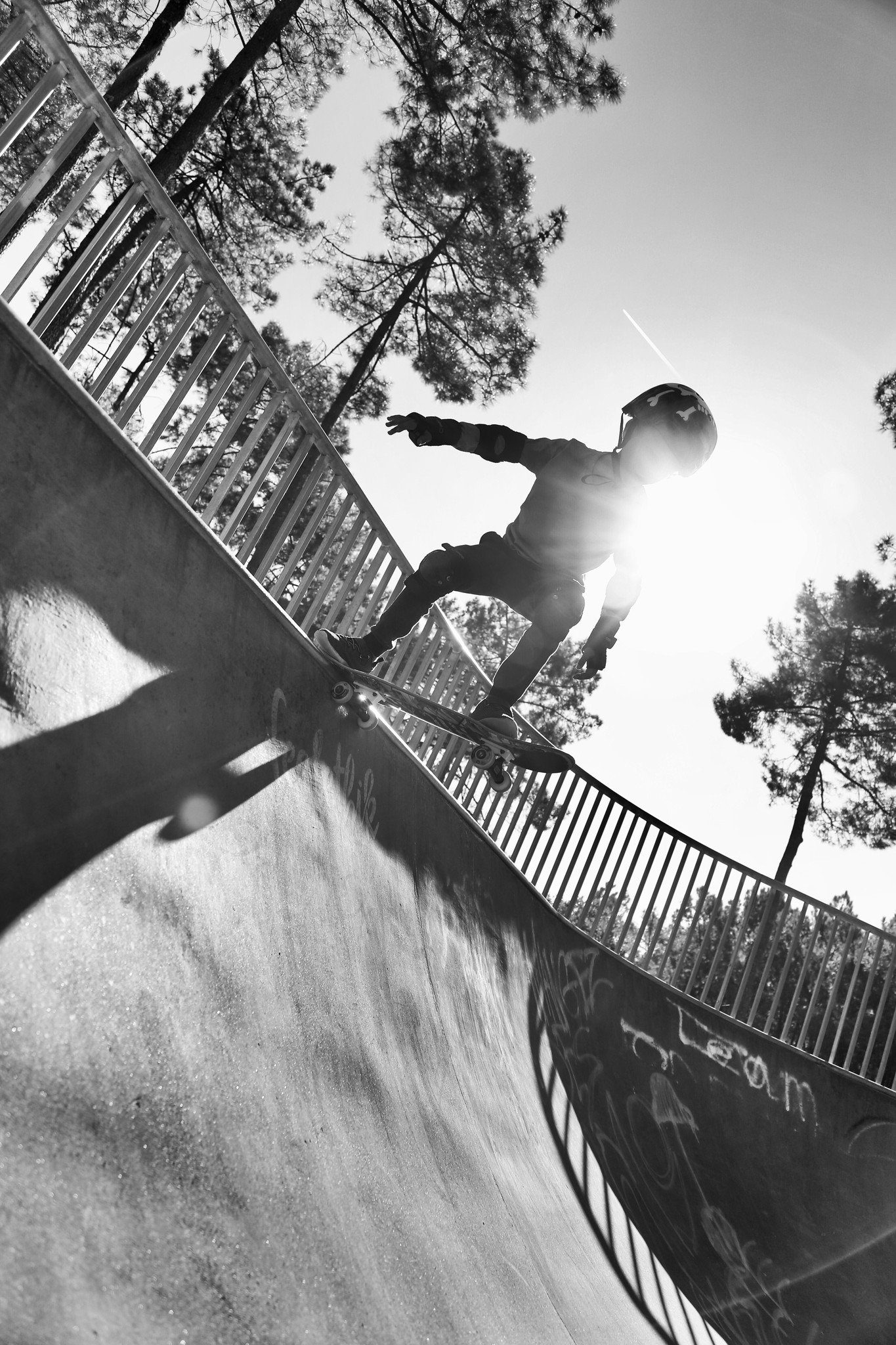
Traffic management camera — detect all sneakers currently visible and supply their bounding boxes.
[469,694,518,739]
[314,629,378,674]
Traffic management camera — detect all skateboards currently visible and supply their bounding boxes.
[312,632,575,793]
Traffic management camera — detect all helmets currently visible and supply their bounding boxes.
[620,384,717,471]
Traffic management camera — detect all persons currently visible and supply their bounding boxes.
[312,382,718,736]
